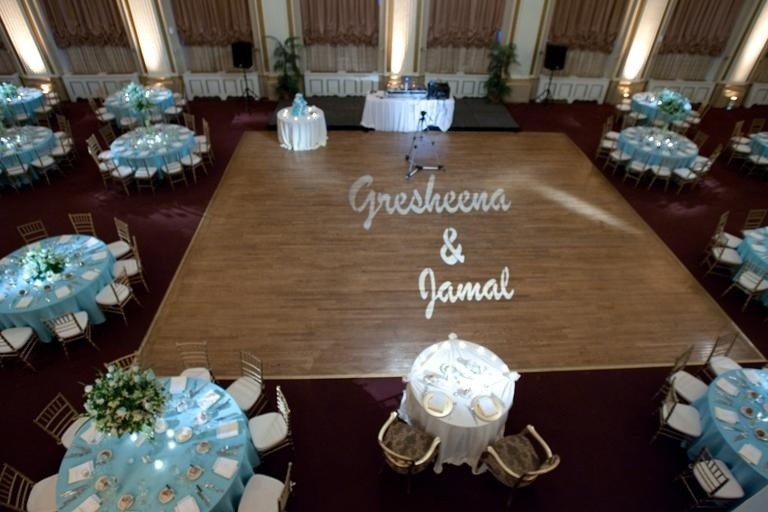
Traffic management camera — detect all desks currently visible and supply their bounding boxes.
[359,89,457,133]
[276,105,328,154]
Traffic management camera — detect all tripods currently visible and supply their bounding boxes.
[236,77,263,105]
[531,70,557,107]
[404,118,445,180]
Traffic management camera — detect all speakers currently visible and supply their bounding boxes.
[230,40,254,69]
[542,43,569,71]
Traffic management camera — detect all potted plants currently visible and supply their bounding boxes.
[483,37,523,107]
[266,34,303,106]
